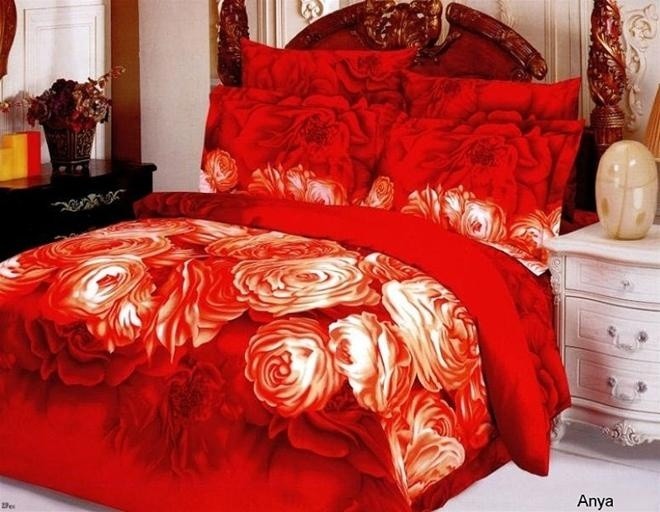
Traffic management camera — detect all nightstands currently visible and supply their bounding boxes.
[0,157,157,261]
[543,221,660,450]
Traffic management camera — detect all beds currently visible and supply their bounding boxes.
[0,0,633,512]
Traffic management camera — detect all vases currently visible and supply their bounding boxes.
[41,123,98,175]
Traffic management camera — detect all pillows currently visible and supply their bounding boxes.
[199,36,586,276]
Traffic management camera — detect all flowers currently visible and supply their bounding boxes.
[27,66,127,123]
